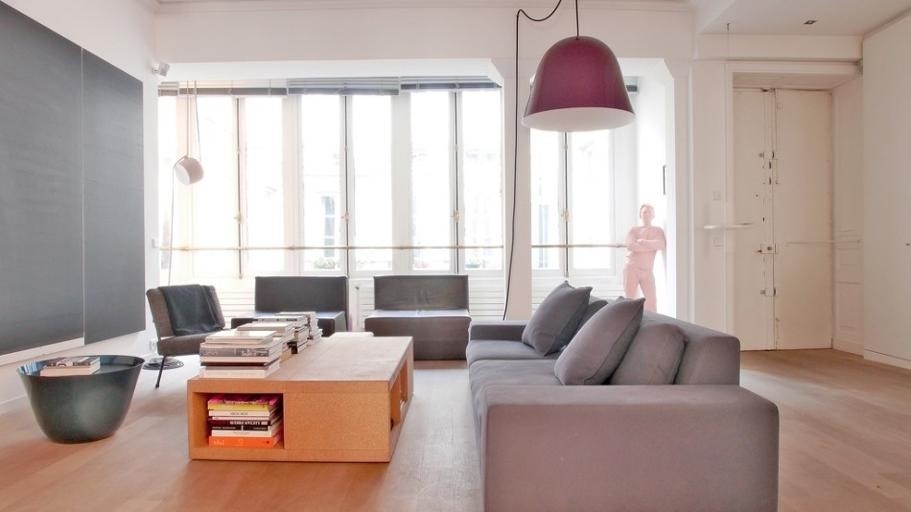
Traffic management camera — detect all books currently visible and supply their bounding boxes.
[207,395,282,448]
[198,310,323,375]
[40,357,101,376]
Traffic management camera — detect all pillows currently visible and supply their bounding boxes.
[518,278,691,385]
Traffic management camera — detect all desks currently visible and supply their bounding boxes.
[17,354,145,442]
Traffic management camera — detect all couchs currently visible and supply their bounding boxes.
[466,299,781,510]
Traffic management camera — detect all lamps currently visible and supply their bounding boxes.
[501,2,636,322]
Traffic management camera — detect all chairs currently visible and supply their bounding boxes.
[231,274,473,363]
[147,283,229,389]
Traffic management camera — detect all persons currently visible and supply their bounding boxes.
[621,204,667,313]
